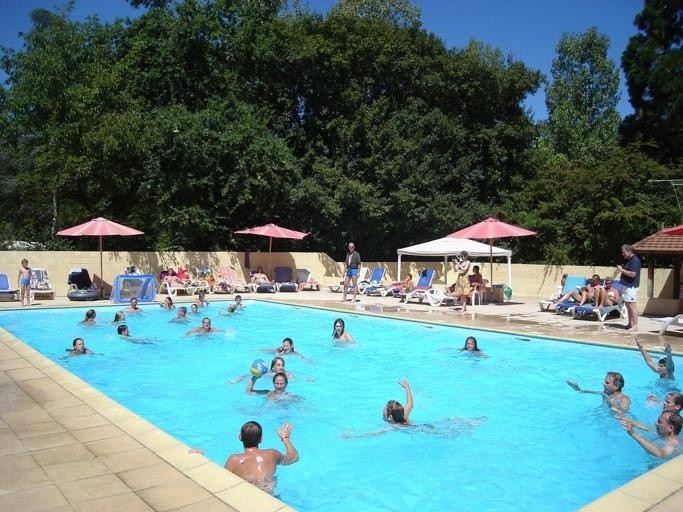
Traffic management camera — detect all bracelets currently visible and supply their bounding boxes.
[635,340,640,342]
[281,436,288,440]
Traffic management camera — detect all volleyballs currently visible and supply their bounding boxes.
[251,359,269,378]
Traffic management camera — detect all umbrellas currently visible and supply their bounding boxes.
[447,216,538,294]
[234,221,310,281]
[53,214,144,287]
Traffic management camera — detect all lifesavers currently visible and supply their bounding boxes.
[67,289,100,301]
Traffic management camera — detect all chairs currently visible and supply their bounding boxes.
[539,275,588,315]
[325,265,489,310]
[572,280,629,324]
[554,278,606,319]
[30,268,53,305]
[157,267,323,298]
[0,273,21,303]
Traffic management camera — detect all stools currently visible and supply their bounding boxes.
[650,312,683,338]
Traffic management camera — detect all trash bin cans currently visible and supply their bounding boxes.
[274,266,292,283]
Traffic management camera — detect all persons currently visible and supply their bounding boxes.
[181,272,190,286]
[112,311,125,323]
[337,241,361,303]
[159,296,175,311]
[194,290,210,307]
[218,304,238,317]
[646,392,682,413]
[232,294,247,308]
[16,258,33,307]
[79,308,97,325]
[248,336,319,367]
[328,318,354,345]
[565,371,631,414]
[553,274,600,307]
[185,419,300,495]
[437,336,493,360]
[250,265,272,285]
[187,303,200,315]
[607,407,681,459]
[226,357,315,385]
[163,267,185,288]
[594,277,619,308]
[123,296,145,313]
[67,338,95,357]
[444,265,482,293]
[117,325,157,344]
[186,316,225,333]
[168,307,190,321]
[386,273,412,290]
[341,379,487,444]
[632,325,675,382]
[243,373,305,408]
[453,251,471,312]
[614,243,640,332]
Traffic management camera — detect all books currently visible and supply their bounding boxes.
[575,285,588,294]
